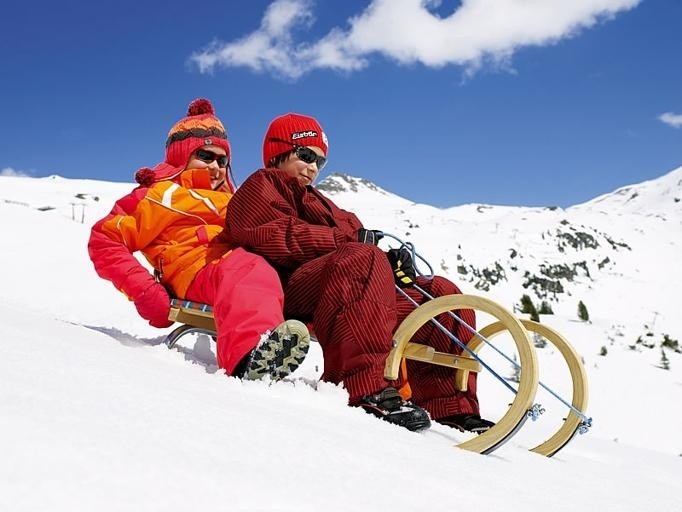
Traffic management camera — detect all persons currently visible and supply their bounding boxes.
[222,111,496,434]
[86,95,313,380]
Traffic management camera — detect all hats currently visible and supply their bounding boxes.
[260,111,329,168]
[134,96,236,196]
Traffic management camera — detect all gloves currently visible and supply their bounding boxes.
[134,287,176,329]
[357,224,383,248]
[386,248,417,288]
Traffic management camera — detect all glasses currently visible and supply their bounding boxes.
[192,147,231,169]
[268,136,328,166]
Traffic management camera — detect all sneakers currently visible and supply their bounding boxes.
[349,386,431,433]
[231,317,312,384]
[435,413,494,432]
